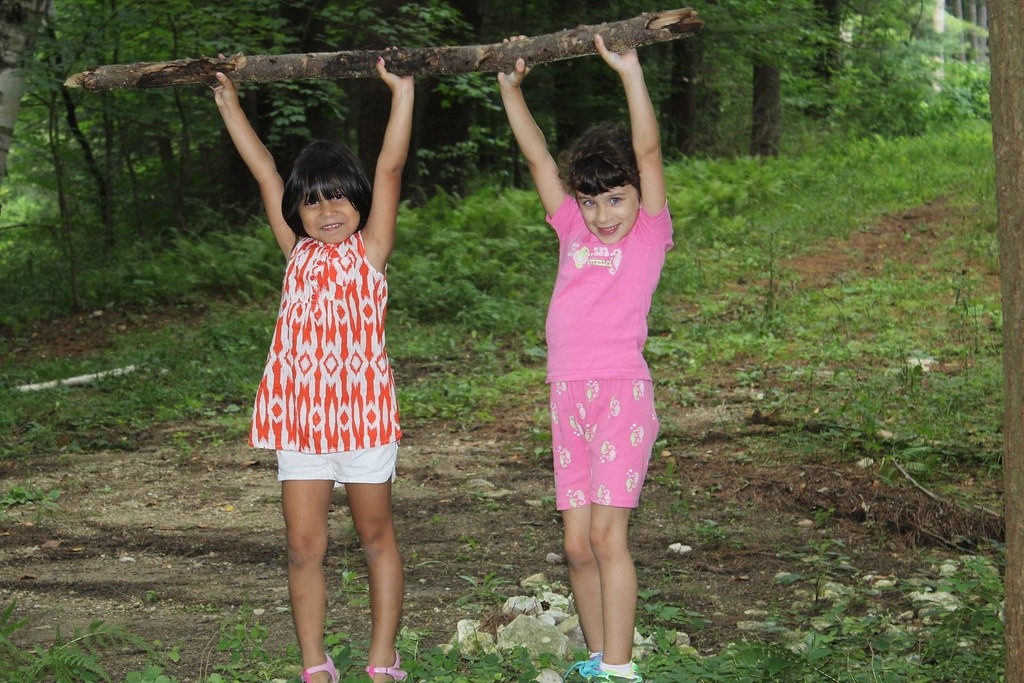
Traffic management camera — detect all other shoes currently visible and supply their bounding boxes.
[586,654,602,662]
[586,662,643,683]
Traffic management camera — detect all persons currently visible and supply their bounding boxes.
[498,32,673,683]
[208,51,414,683]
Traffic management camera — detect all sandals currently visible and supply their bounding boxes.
[301,653,340,683]
[365,650,408,683]
